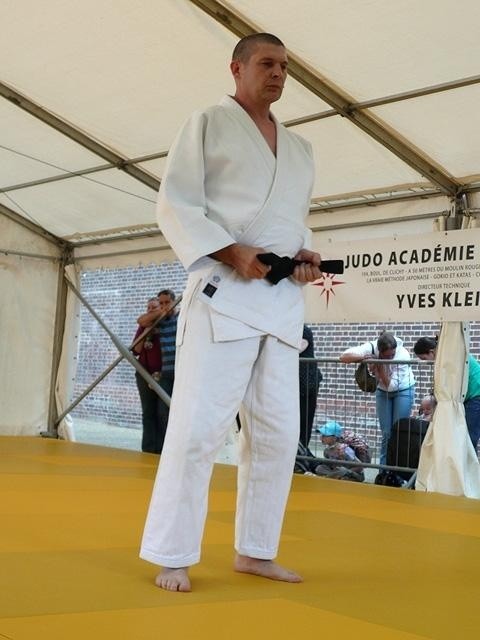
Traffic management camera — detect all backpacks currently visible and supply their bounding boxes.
[336,431,372,465]
[375,468,401,487]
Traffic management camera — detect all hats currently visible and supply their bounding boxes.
[317,421,343,438]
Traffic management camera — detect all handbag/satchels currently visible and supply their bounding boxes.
[355,361,377,393]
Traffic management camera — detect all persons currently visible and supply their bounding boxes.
[130,292,181,453]
[139,33,323,591]
[299,326,479,487]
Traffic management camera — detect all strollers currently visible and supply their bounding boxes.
[374,415,431,490]
